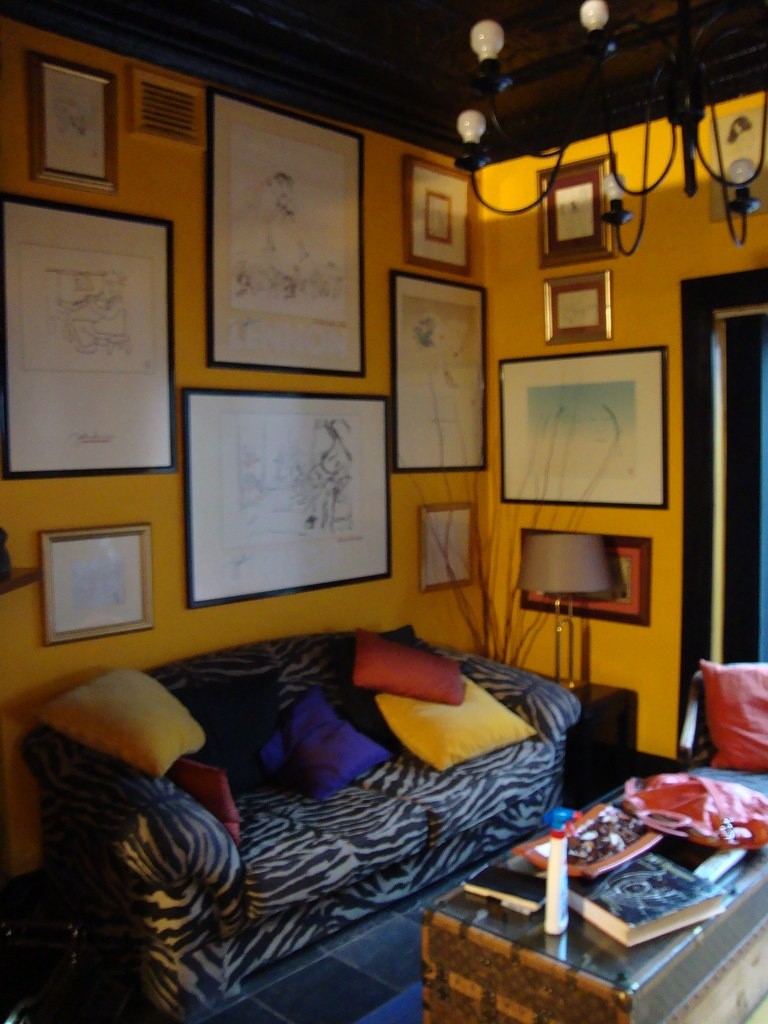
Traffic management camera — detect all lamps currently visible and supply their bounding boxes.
[457,0,768,257]
[517,520,617,693]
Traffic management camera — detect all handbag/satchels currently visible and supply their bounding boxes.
[625,772,768,850]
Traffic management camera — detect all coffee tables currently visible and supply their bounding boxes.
[422,764,768,1024]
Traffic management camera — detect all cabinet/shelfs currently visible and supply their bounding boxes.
[540,673,638,810]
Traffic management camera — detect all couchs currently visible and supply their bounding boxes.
[20,623,580,1018]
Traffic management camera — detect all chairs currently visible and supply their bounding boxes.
[678,668,713,771]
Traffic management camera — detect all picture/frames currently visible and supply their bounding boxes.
[22,52,123,197]
[387,268,490,476]
[415,502,477,593]
[519,528,653,629]
[37,524,155,647]
[200,87,367,379]
[0,190,176,482]
[179,386,392,611]
[404,156,475,279]
[540,271,610,345]
[532,155,615,271]
[496,347,672,510]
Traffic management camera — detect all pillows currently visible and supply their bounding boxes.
[373,676,538,775]
[701,659,768,773]
[29,664,209,780]
[172,758,241,849]
[350,629,465,708]
[254,690,391,803]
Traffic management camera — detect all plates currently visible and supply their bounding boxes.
[512,800,663,880]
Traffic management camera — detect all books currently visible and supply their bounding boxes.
[463,865,548,916]
[569,849,726,948]
